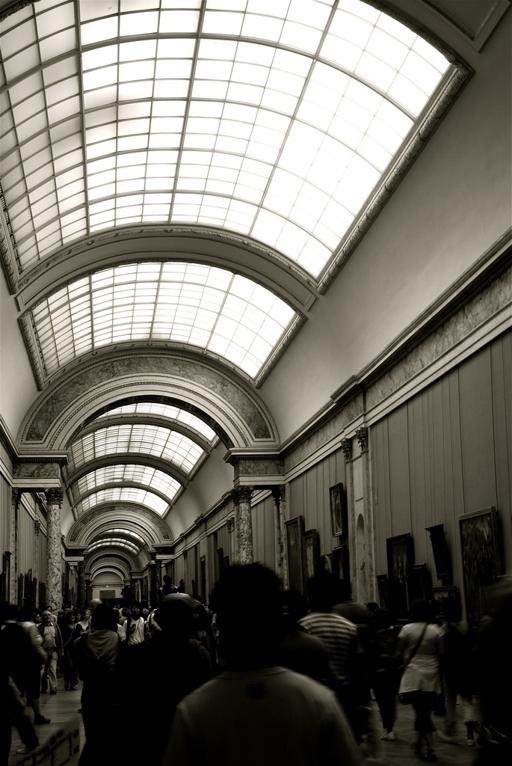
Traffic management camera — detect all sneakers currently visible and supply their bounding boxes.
[41,687,77,694]
[380,711,512,759]
[33,715,50,726]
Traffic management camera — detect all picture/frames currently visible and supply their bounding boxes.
[385,532,416,628]
[285,515,320,596]
[458,505,502,632]
[328,481,347,537]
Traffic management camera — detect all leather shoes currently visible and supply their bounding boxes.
[16,748,27,754]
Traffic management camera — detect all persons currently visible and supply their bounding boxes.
[57,604,160,705]
[162,563,363,766]
[2,604,64,766]
[77,592,212,766]
[279,562,511,760]
